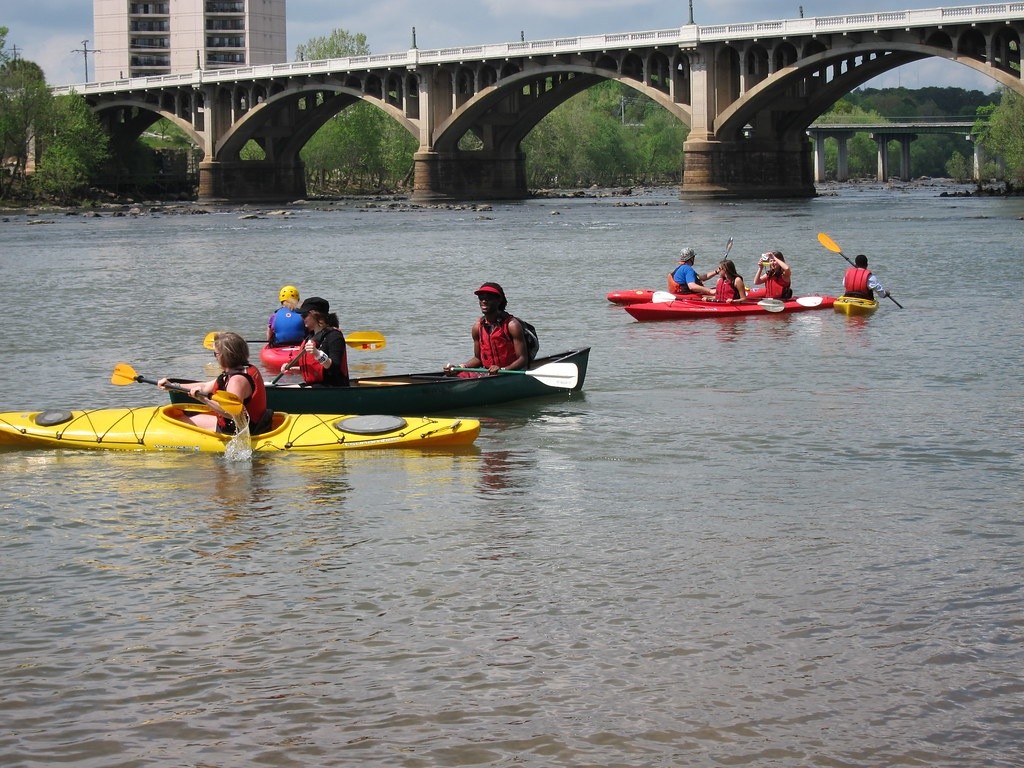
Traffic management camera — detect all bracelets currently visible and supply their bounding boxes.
[500,367,506,370]
[458,363,467,368]
[715,270,719,274]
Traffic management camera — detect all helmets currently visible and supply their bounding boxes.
[279,285,301,303]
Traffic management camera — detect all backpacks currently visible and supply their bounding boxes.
[477,312,539,361]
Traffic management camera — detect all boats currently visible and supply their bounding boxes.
[832,295,879,318]
[606,287,707,303]
[0,408,484,454]
[154,346,596,415]
[623,296,838,321]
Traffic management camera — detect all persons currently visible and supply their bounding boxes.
[753,251,791,298]
[157,332,266,436]
[443,282,530,378]
[701,259,746,303]
[267,286,310,348]
[278,297,349,387]
[842,254,890,300]
[668,247,722,294]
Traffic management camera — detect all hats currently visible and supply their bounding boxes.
[296,297,329,313]
[474,285,503,299]
[679,247,697,261]
[204,331,220,350]
